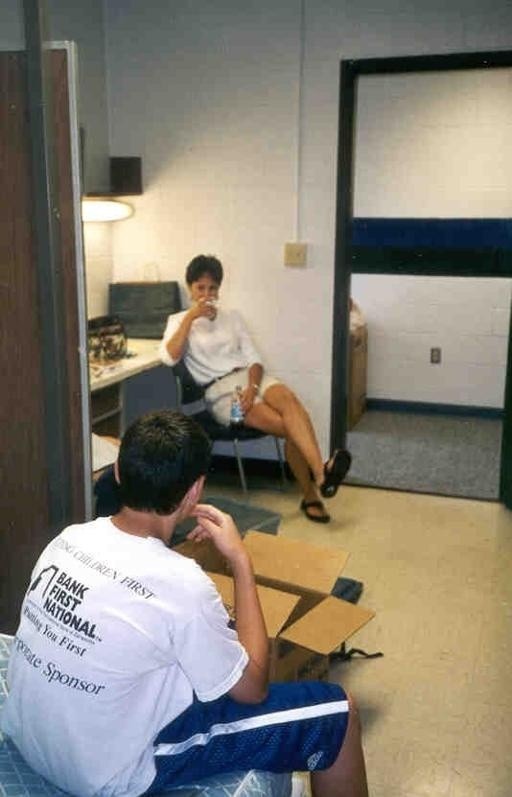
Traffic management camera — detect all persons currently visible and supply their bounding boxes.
[159,254,351,524]
[0,410,369,796]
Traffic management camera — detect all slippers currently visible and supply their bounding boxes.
[301,448,352,523]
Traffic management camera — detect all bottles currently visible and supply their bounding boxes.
[228,385,245,426]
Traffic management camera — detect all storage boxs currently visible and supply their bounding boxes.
[167,527,375,679]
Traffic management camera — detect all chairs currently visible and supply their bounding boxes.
[173,360,288,494]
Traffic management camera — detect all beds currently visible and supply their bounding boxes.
[0,633,293,796]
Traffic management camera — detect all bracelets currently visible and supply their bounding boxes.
[250,382,259,392]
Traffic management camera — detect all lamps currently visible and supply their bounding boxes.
[81,198,132,223]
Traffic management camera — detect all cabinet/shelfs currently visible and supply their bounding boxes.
[90,336,163,484]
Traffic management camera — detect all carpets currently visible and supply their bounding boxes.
[341,408,502,501]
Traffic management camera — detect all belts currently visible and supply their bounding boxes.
[203,366,243,390]
